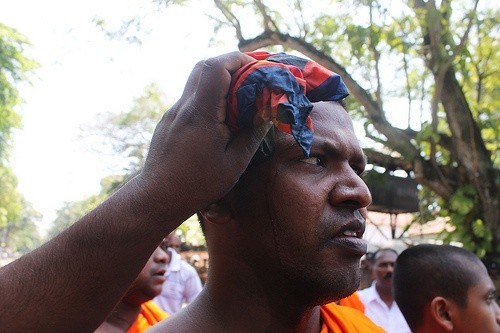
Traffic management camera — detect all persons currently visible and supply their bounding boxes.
[139,52,390,333]
[0,237,500,333]
[0,50,274,333]
